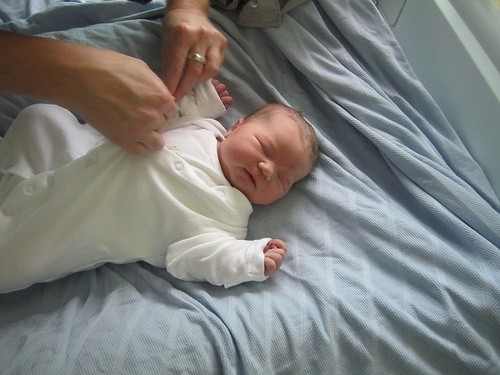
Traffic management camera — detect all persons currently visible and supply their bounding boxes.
[0,77,320,293]
[0,0,229,156]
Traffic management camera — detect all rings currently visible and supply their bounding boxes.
[188,52,208,66]
